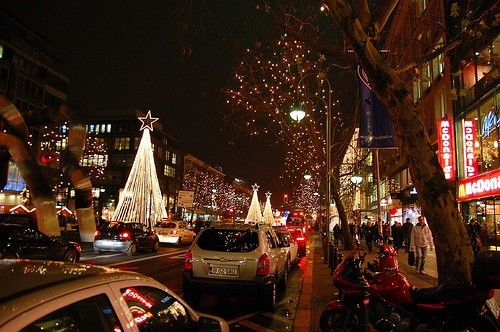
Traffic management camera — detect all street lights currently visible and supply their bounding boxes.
[94,187,106,222]
[290,72,331,265]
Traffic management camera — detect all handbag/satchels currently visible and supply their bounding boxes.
[408,250,415,265]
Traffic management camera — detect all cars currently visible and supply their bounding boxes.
[0,259,230,332]
[153,218,197,245]
[270,225,307,264]
[92,222,161,256]
[285,218,315,235]
[191,219,252,226]
[0,223,82,263]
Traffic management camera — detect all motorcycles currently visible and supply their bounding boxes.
[319,244,500,332]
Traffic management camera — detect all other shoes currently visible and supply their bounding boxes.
[416,270,419,273]
[420,270,425,274]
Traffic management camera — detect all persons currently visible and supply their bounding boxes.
[331,217,413,253]
[466,218,483,255]
[410,216,434,275]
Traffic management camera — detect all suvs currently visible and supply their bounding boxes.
[183,225,290,309]
